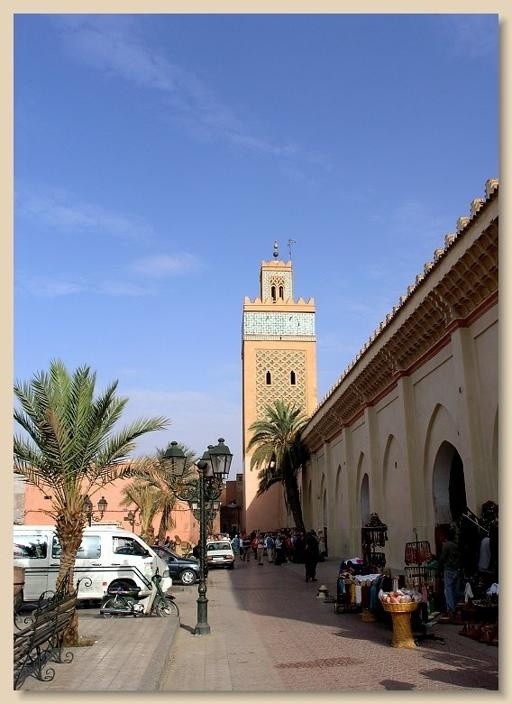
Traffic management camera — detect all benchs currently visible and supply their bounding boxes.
[14,577,93,689]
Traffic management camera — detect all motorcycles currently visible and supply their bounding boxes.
[96,568,182,616]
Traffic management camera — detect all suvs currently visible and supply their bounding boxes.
[116,542,208,586]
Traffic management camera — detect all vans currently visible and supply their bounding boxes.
[13,522,174,605]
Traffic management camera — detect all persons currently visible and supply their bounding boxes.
[174,535,182,558]
[300,531,320,583]
[192,538,201,562]
[427,526,461,619]
[212,528,327,569]
[165,535,171,548]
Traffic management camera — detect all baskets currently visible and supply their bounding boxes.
[381,602,418,613]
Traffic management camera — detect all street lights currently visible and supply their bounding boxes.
[127,510,135,533]
[160,433,237,635]
[80,494,109,528]
